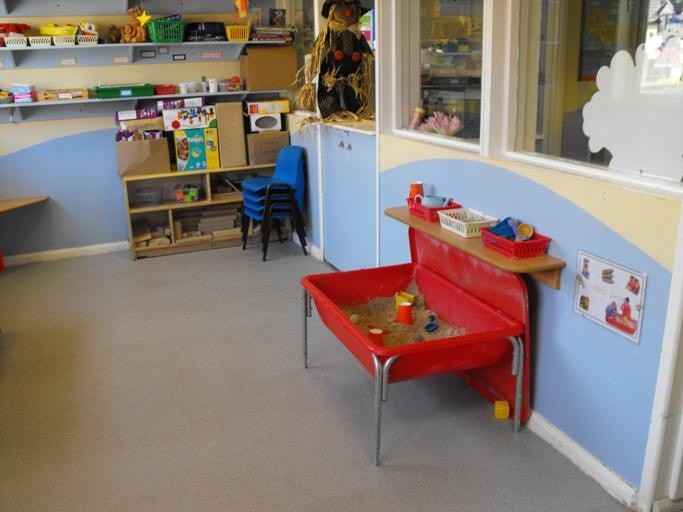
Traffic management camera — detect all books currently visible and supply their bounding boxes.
[252,24,293,41]
[173,202,243,244]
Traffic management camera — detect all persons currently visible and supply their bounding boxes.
[119,23,136,42]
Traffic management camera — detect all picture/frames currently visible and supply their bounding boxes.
[577,0,620,82]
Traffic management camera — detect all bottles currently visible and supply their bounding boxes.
[410,107,426,130]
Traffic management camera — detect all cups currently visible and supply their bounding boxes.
[517,223,534,240]
[408,181,422,200]
[394,302,412,324]
[368,328,384,347]
[178,81,196,96]
[209,79,218,94]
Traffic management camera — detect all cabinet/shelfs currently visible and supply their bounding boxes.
[421,33,481,139]
[122,163,293,261]
[0,12,287,123]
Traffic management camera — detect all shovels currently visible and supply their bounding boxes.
[424,313,438,331]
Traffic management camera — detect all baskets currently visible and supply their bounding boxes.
[437,208,500,238]
[4,35,99,47]
[146,19,185,43]
[225,25,251,42]
[479,226,551,259]
[406,197,461,223]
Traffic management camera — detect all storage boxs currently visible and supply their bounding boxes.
[172,128,221,172]
[215,101,248,169]
[115,137,171,179]
[240,44,301,91]
[246,130,292,166]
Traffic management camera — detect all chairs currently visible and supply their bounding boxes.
[240,144,308,262]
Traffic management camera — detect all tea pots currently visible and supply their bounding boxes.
[413,193,456,208]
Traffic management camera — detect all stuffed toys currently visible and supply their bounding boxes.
[290,0,376,124]
